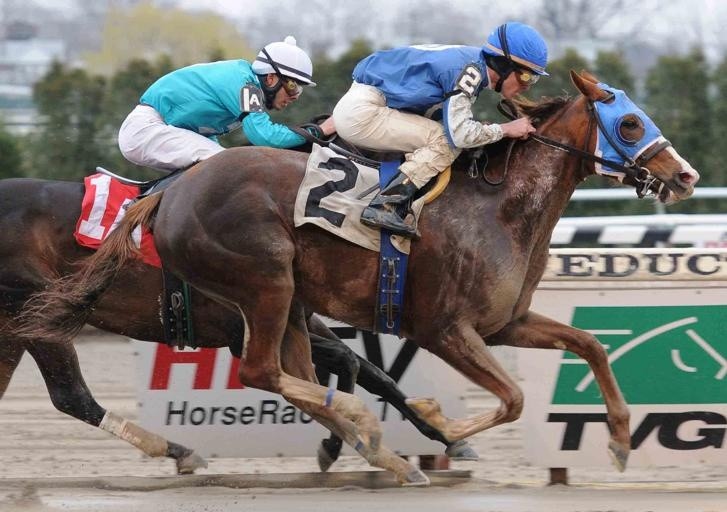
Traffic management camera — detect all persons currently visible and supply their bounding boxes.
[333,21,548,234]
[117,36,336,222]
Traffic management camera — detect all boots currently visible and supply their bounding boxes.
[122,169,187,233]
[360,170,422,242]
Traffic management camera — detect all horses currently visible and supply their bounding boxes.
[0,135,481,477]
[6,68,700,488]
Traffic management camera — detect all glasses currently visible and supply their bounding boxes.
[281,78,302,96]
[515,68,541,86]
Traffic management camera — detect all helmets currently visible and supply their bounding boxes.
[252,35,317,87]
[482,22,551,77]
